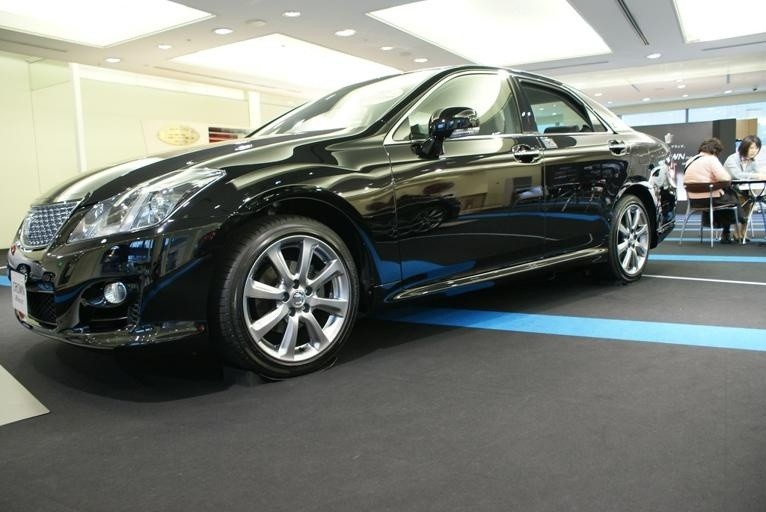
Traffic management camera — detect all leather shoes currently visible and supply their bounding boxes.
[742,230,750,241]
[733,231,743,241]
[740,216,748,224]
[721,231,732,244]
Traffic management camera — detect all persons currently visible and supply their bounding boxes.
[687,136,748,243]
[724,135,762,242]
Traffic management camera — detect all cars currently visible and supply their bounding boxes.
[7,64,677,379]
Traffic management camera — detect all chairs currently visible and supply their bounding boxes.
[678,180,742,247]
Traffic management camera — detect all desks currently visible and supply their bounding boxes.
[731,180,766,245]
